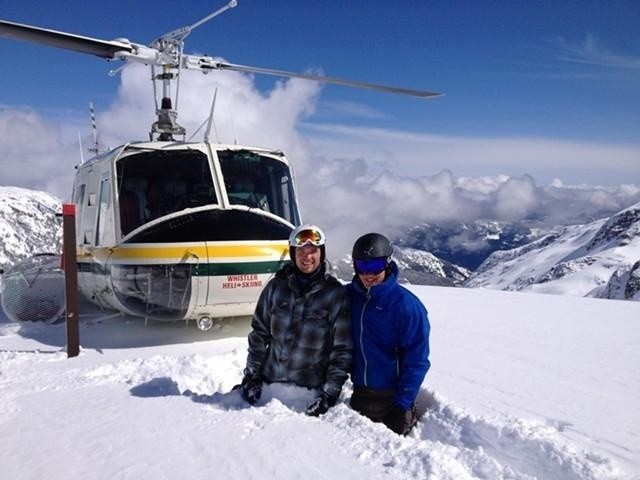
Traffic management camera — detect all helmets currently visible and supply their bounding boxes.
[352,233,393,274]
[289,224,326,248]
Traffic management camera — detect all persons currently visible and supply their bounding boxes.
[241,223,353,416]
[345,232,430,434]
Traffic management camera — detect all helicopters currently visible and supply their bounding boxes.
[0,2,447,333]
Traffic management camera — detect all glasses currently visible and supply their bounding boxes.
[354,257,387,275]
[294,230,321,245]
